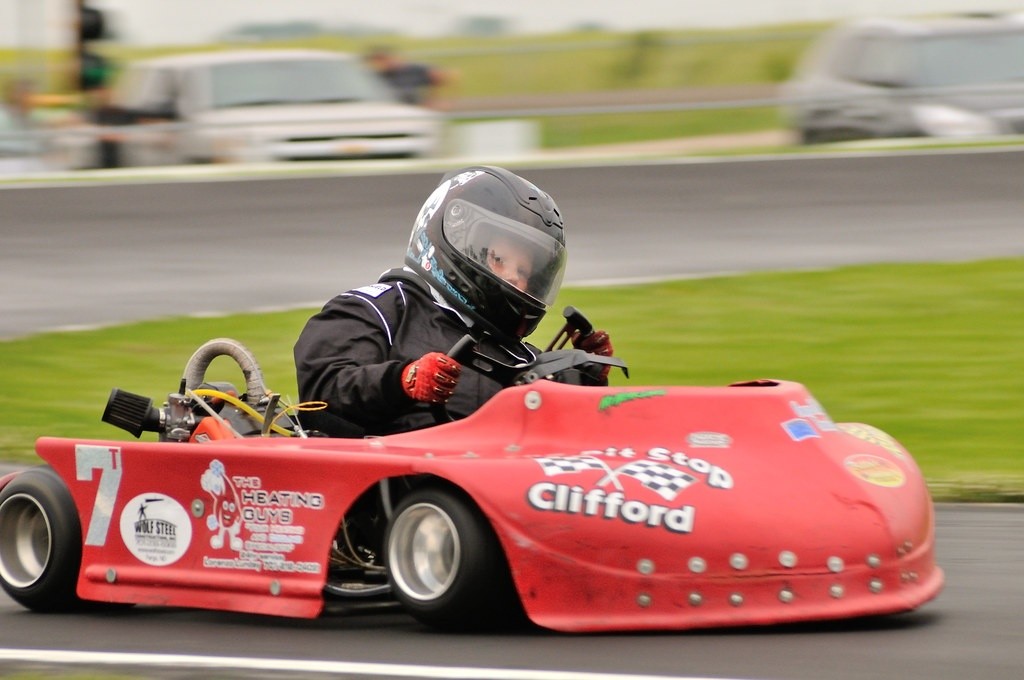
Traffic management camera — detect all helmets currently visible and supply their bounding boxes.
[405,165,564,346]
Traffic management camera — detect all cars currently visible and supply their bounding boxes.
[780,8,1024,145]
[106,52,445,160]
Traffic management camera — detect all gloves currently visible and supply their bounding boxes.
[401,352,463,404]
[571,331,613,381]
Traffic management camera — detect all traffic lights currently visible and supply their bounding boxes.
[75,7,108,88]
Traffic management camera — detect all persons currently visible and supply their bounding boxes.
[293,165,613,437]
[28,0,184,170]
[362,45,465,111]
